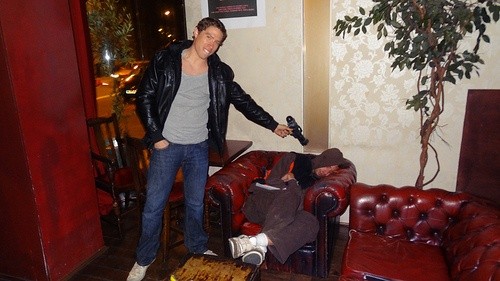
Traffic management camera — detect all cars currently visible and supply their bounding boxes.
[111,60,151,85]
[118,64,145,104]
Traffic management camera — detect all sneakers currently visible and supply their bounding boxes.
[127,257,156,281]
[228,234,256,258]
[242,247,265,267]
[203,249,218,256]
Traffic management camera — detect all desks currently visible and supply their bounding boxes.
[163,253,262,281]
[208,141,250,168]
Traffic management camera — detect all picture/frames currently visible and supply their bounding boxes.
[200,0,266,30]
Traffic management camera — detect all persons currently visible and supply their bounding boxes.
[228,147,344,267]
[125,16,294,281]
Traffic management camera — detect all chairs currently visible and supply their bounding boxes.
[85,113,144,244]
[123,134,211,259]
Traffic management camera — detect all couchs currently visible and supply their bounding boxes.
[204,150,357,281]
[349,184,500,281]
